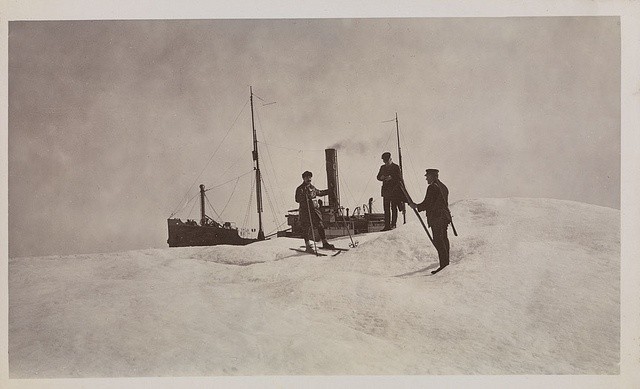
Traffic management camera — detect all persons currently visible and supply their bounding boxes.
[295,171,334,254]
[377,152,401,231]
[409,169,452,274]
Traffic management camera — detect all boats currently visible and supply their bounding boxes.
[156,86,409,247]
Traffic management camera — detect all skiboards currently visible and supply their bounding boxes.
[291,247,348,256]
[300,245,349,252]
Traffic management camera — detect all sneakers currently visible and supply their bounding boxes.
[306,245,314,254]
[323,242,335,250]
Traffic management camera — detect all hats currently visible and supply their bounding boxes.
[425,169,439,176]
[382,152,391,159]
[302,171,312,178]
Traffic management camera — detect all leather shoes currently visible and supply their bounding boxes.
[380,226,391,231]
[431,265,445,274]
[390,225,396,230]
